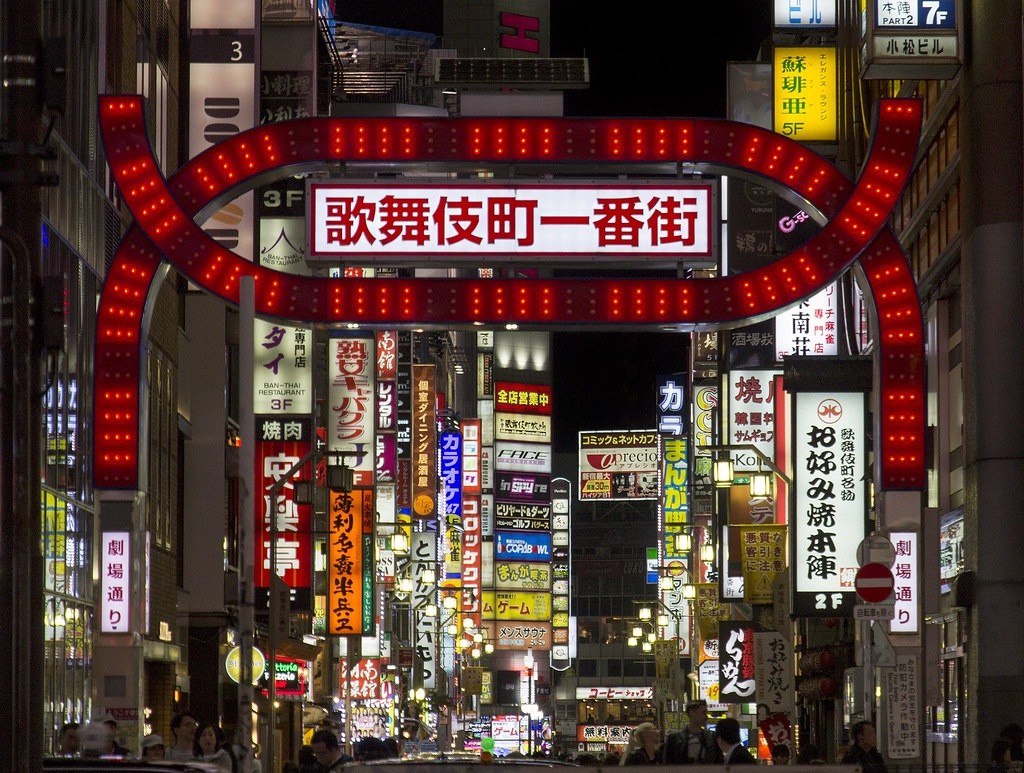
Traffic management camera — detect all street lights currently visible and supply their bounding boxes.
[523,655,534,759]
[261,449,368,773]
[412,586,500,755]
[695,443,798,767]
[626,598,680,766]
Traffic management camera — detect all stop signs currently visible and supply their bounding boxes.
[854,562,895,604]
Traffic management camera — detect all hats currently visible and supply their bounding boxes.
[96,712,116,727]
[141,735,164,748]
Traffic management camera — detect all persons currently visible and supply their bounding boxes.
[607,714,613,721]
[842,721,887,773]
[620,722,660,767]
[165,712,198,758]
[800,743,826,766]
[772,744,789,765]
[716,718,757,765]
[60,723,82,754]
[193,721,238,773]
[531,751,621,766]
[77,721,108,754]
[97,714,127,755]
[991,725,1024,773]
[283,730,399,773]
[662,699,724,764]
[141,735,165,759]
[588,714,594,722]
